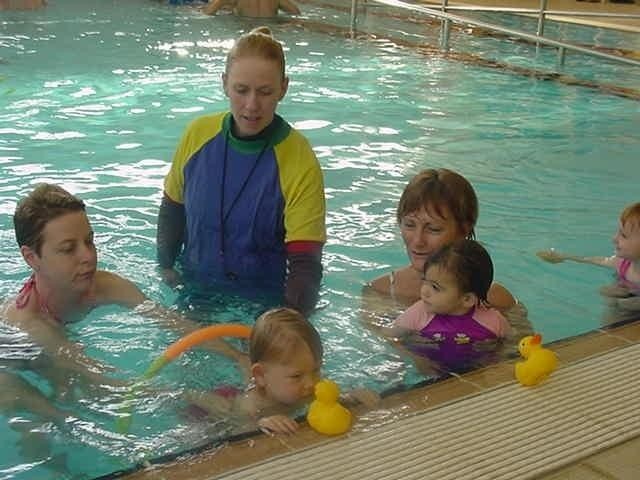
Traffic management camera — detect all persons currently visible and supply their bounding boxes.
[186,307,383,436]
[0,183,251,479]
[535,202,640,324]
[156,26,328,321]
[204,0,302,16]
[360,168,536,364]
[382,239,515,381]
